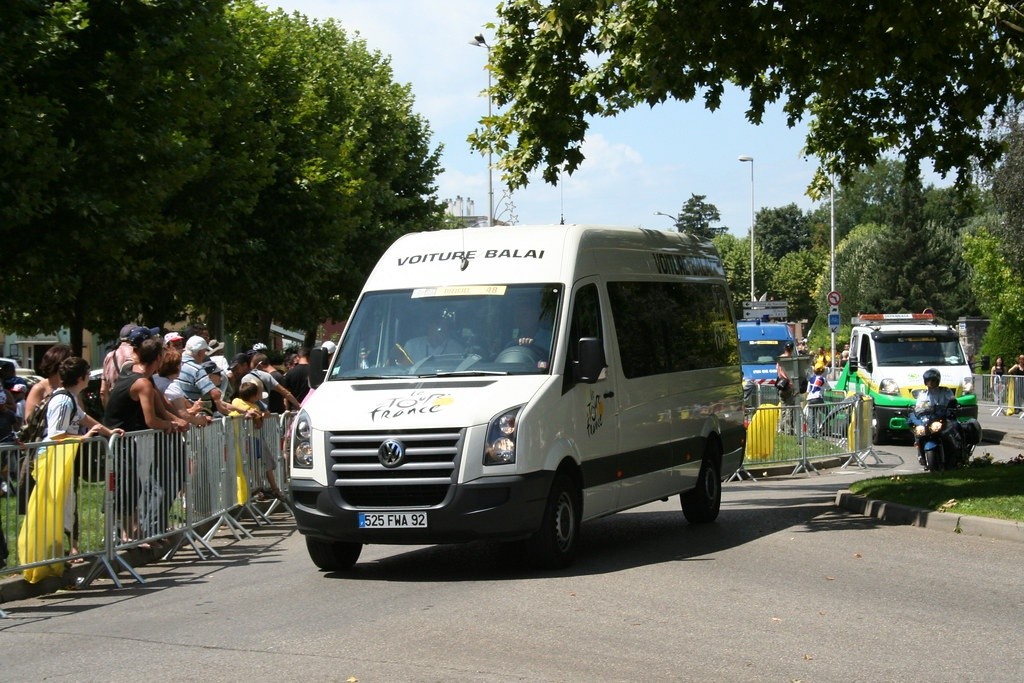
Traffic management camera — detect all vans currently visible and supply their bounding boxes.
[287,223,749,573]
[736,320,798,406]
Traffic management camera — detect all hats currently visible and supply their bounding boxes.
[322,341,337,354]
[203,362,224,376]
[205,339,226,356]
[119,324,137,341]
[128,326,160,342]
[163,332,184,344]
[785,341,795,348]
[229,354,249,371]
[815,364,824,371]
[184,335,212,352]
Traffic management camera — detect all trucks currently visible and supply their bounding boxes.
[822,313,980,444]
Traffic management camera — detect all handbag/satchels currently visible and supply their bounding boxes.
[775,377,789,389]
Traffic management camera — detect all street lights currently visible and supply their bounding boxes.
[739,156,754,308]
[468,31,492,227]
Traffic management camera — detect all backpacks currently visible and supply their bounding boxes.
[17,390,76,460]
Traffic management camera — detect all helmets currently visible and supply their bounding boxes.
[923,369,941,386]
[253,343,267,350]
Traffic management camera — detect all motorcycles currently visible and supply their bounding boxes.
[907,398,984,474]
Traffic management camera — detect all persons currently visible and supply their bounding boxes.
[990,354,1007,410]
[101,322,378,549]
[494,300,581,382]
[1008,354,1024,414]
[796,341,850,374]
[775,341,798,436]
[0,342,126,563]
[806,366,831,439]
[916,370,962,462]
[405,306,465,369]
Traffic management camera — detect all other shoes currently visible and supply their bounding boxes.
[265,486,288,502]
